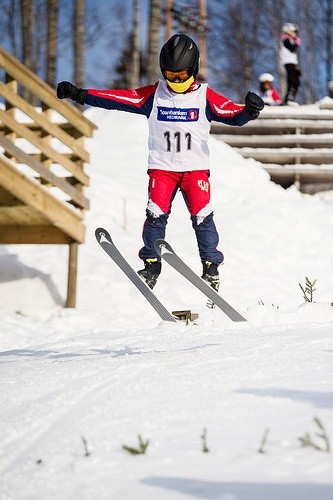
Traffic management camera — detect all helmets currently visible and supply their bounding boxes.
[282,23,299,38]
[257,72,274,83]
[159,33,200,94]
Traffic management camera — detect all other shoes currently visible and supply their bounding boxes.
[285,97,299,105]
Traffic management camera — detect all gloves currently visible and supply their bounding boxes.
[294,37,302,45]
[57,81,88,105]
[245,91,265,116]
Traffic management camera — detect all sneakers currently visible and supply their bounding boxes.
[136,258,162,289]
[200,259,220,292]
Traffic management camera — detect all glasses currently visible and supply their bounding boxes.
[164,69,191,80]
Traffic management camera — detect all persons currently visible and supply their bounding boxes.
[57,34,264,290]
[279,22,303,106]
[253,73,284,106]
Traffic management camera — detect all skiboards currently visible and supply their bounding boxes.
[93,225,244,323]
